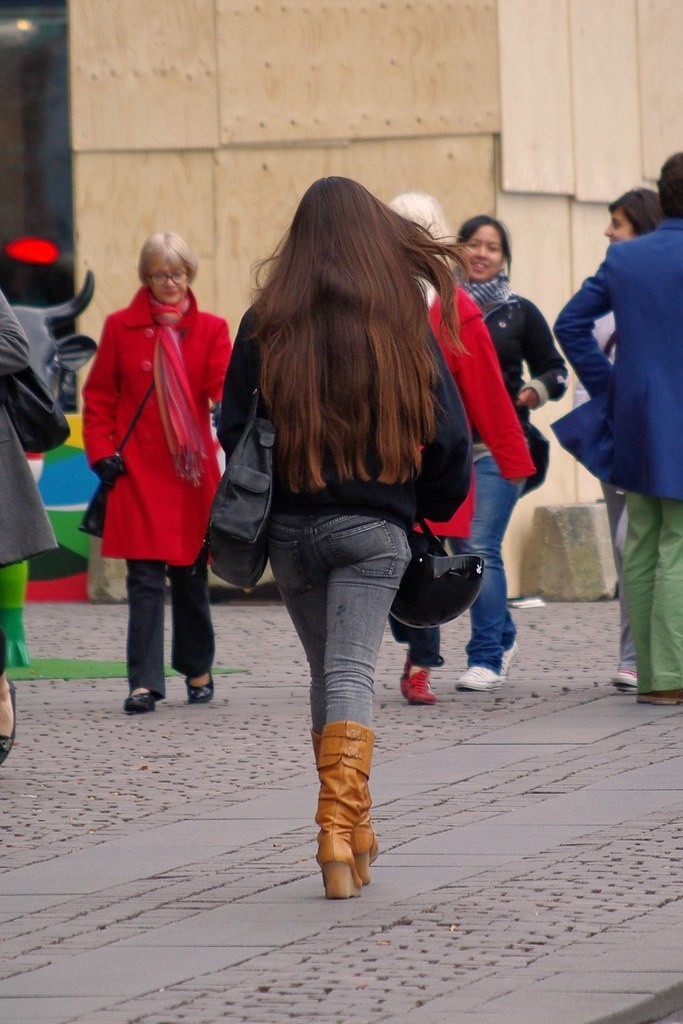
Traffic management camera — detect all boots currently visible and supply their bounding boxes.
[316,721,376,899]
[310,726,378,887]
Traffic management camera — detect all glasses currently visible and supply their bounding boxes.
[145,270,190,286]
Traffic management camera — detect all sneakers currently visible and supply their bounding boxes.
[400,657,409,698]
[455,667,502,691]
[500,642,518,678]
[409,672,436,704]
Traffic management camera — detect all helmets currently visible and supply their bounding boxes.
[388,551,486,629]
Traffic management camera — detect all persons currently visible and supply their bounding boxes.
[220,177,472,899]
[82,233,231,715]
[551,153,683,707]
[389,213,568,708]
[0,286,59,770]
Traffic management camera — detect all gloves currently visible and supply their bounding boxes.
[93,455,126,485]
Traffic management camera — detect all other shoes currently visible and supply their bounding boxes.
[183,673,213,703]
[612,670,637,692]
[124,692,155,712]
[637,689,683,705]
[0,680,16,766]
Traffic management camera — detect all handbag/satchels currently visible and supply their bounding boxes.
[207,418,277,592]
[78,482,108,538]
